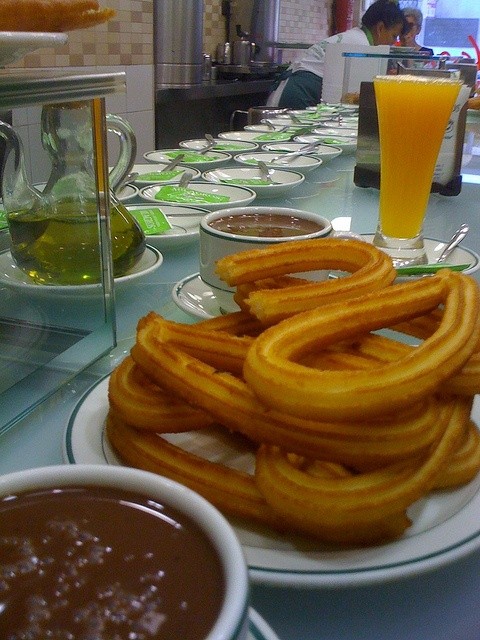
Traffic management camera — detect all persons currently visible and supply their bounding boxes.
[386,8,434,74]
[265,0,408,109]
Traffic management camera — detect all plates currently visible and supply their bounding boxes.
[294,135,358,154]
[139,181,257,208]
[276,114,333,121]
[286,109,339,117]
[202,167,305,198]
[352,233,479,282]
[314,127,358,136]
[106,163,201,183]
[65,316,480,589]
[32,183,137,200]
[306,106,342,113]
[261,119,320,126]
[261,142,343,162]
[119,202,213,251]
[244,125,302,133]
[336,116,358,121]
[324,103,359,108]
[391,46,413,53]
[324,121,358,128]
[180,138,259,153]
[234,152,322,175]
[235,605,278,639]
[217,131,291,144]
[171,270,342,320]
[0,243,164,303]
[2,32,68,65]
[143,148,231,168]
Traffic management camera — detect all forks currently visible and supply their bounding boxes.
[257,160,283,184]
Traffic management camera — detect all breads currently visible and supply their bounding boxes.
[106,237,479,554]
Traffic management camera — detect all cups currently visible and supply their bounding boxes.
[372,75,462,270]
[201,53,212,81]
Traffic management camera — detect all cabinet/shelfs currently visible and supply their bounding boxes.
[1,59,127,436]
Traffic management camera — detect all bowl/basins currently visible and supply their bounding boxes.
[0,464,248,639]
[201,207,332,316]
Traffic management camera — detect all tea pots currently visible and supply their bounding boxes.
[233,40,255,67]
[216,42,231,64]
[230,107,291,131]
[0,95,145,285]
